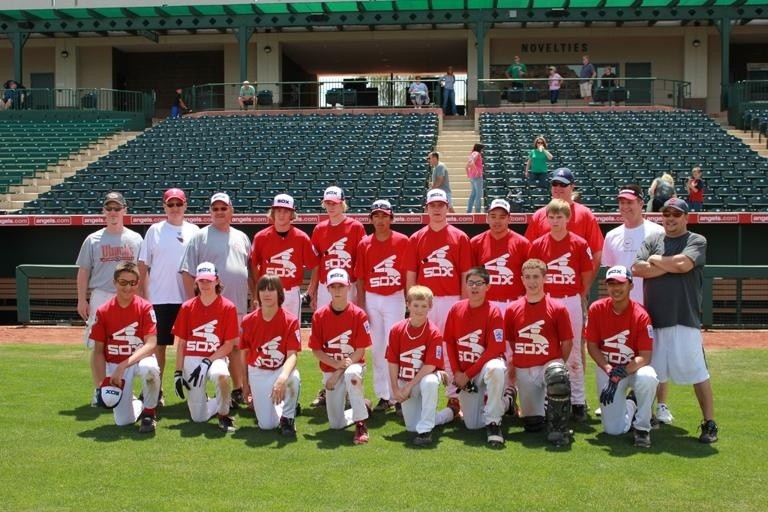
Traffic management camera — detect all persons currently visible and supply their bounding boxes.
[307,268,372,444]
[240,273,300,439]
[442,267,519,444]
[170,87,193,118]
[547,66,564,104]
[470,197,531,386]
[523,168,604,374]
[385,285,460,445]
[586,265,659,449]
[504,258,573,433]
[137,187,201,408]
[238,81,258,110]
[596,184,674,426]
[526,198,592,424]
[631,197,719,444]
[306,185,367,406]
[354,200,408,415]
[645,173,675,212]
[170,261,238,431]
[504,55,528,88]
[177,192,249,409]
[75,192,152,407]
[408,76,428,109]
[525,137,553,180]
[438,65,459,116]
[429,151,455,213]
[465,143,485,213]
[251,193,320,417]
[687,167,706,211]
[0,81,27,109]
[579,55,596,102]
[89,261,161,434]
[406,188,472,400]
[601,66,619,89]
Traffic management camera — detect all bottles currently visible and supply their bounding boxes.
[539,147,543,153]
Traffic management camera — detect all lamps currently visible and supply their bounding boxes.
[693,39,702,47]
[263,44,272,54]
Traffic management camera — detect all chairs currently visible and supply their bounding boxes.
[478,85,767,212]
[1,86,440,214]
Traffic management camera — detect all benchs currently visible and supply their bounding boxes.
[1,275,315,320]
[596,277,768,324]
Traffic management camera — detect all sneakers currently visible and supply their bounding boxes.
[158,389,165,407]
[280,416,296,436]
[447,398,461,419]
[696,419,718,443]
[633,429,651,447]
[413,433,433,445]
[553,433,570,445]
[91,387,99,408]
[138,416,157,432]
[311,389,326,405]
[394,402,402,417]
[230,398,241,409]
[486,422,505,444]
[374,399,392,411]
[296,403,300,415]
[353,420,369,444]
[594,407,601,415]
[651,414,659,430]
[504,386,518,418]
[364,398,372,415]
[572,404,587,422]
[231,388,251,403]
[656,407,674,424]
[218,414,237,432]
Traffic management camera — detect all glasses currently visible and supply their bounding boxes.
[548,69,553,71]
[427,158,431,160]
[662,210,683,217]
[370,204,392,210]
[104,206,124,212]
[537,142,544,144]
[551,182,572,187]
[212,207,228,211]
[116,279,138,286]
[165,203,183,207]
[466,280,486,286]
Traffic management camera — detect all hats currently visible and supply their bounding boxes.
[322,185,345,204]
[195,261,218,283]
[163,187,186,204]
[371,199,393,216]
[427,188,448,206]
[488,199,510,214]
[548,66,556,71]
[617,187,643,202]
[96,377,125,409]
[272,193,296,211]
[659,198,690,213]
[103,192,127,208]
[551,168,575,184]
[326,269,351,287]
[605,265,633,283]
[210,192,232,207]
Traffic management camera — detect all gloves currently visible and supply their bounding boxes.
[300,292,311,306]
[188,358,211,388]
[174,370,190,400]
[456,380,478,394]
[600,375,621,406]
[606,365,628,378]
